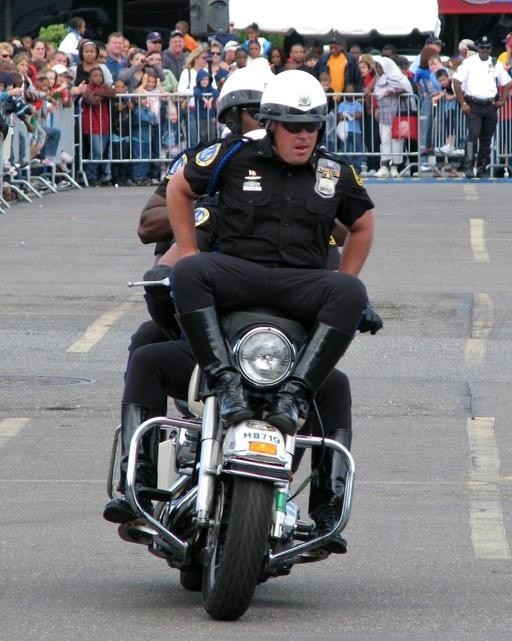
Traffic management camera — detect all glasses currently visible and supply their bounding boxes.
[479,46,491,49]
[2,54,12,58]
[210,52,221,55]
[232,106,260,120]
[281,122,322,134]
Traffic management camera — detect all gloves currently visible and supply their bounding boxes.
[143,264,177,295]
[357,307,383,337]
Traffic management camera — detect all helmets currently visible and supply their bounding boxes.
[254,69,330,123]
[215,65,276,124]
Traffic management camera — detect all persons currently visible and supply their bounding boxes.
[103,68,384,553]
[164,71,375,428]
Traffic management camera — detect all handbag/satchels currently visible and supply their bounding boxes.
[391,115,418,140]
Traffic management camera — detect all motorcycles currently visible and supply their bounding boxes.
[109,278,386,620]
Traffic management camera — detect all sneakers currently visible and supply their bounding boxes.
[3,150,76,201]
[356,141,512,178]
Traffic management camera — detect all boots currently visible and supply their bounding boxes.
[308,427,353,555]
[265,320,355,437]
[101,401,161,524]
[174,305,254,425]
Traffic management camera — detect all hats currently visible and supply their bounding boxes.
[145,29,184,42]
[425,36,446,47]
[51,64,68,75]
[327,35,343,44]
[474,35,494,47]
[223,39,242,53]
